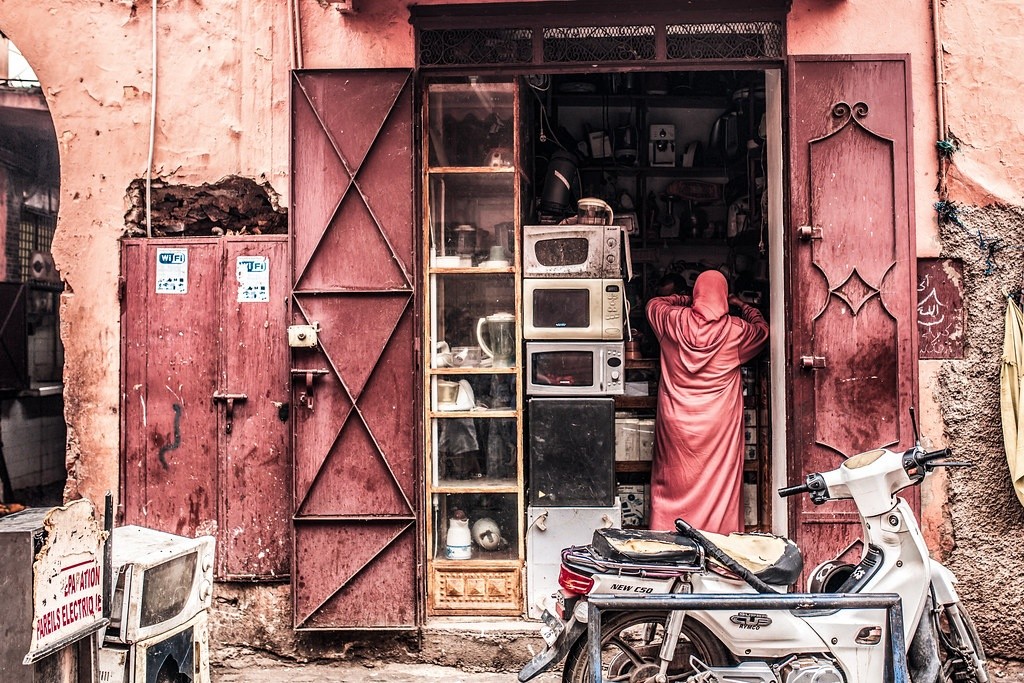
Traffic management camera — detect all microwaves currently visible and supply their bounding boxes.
[99,609,211,683]
[103,524,215,645]
[523,278,632,342]
[523,225,634,282]
[526,343,625,397]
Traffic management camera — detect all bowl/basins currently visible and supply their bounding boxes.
[451,347,481,367]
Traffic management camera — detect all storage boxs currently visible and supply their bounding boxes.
[624,380,649,397]
[615,418,640,462]
[639,419,657,461]
[742,409,757,427]
[743,427,757,445]
[644,485,651,526]
[745,445,757,460]
[617,484,644,527]
[527,497,622,622]
[529,396,616,508]
[744,483,759,526]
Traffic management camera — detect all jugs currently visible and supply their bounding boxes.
[445,518,472,560]
[577,197,614,225]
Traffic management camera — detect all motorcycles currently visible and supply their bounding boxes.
[518,407,988,683]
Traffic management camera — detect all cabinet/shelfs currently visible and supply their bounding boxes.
[423,84,523,621]
[614,359,772,536]
[546,93,756,341]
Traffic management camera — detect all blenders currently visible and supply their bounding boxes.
[476,312,515,368]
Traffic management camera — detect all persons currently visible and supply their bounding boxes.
[645,270,769,536]
